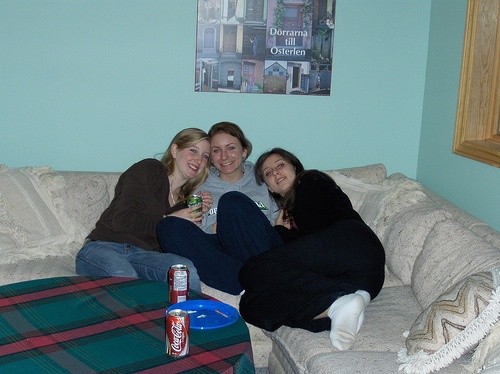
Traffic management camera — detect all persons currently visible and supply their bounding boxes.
[157,121,280,296]
[75,128,213,294]
[239,148,385,350]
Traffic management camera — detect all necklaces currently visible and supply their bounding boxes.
[169,179,176,206]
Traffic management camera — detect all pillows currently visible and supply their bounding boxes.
[398,271,499,373]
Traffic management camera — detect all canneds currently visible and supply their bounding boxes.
[168,264,190,304]
[187,195,202,223]
[165,309,189,357]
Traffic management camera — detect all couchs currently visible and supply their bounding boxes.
[1,163,500,373]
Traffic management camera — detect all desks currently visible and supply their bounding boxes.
[0,276,256,374]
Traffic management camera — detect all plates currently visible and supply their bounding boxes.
[168,300,239,329]
